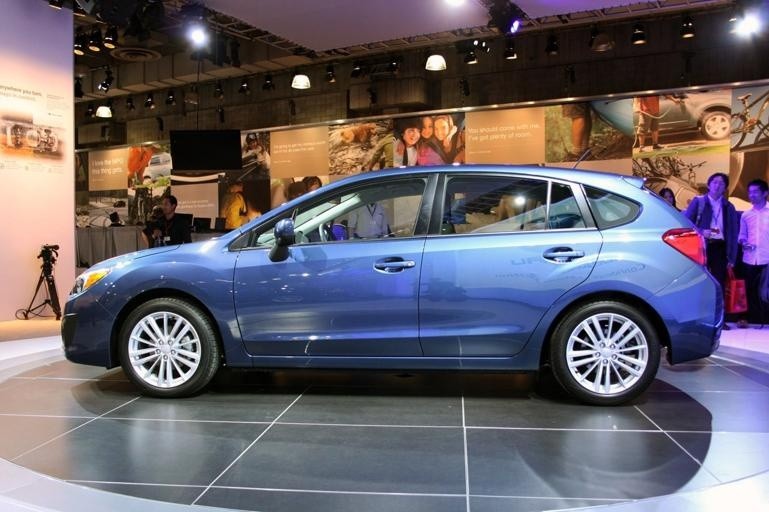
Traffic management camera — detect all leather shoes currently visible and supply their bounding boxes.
[722,320,748,330]
[653,144,664,149]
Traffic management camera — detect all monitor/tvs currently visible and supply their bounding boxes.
[168,129,244,172]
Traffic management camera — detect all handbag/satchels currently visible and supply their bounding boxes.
[725,265,747,313]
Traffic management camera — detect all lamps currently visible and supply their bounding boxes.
[544,42,559,54]
[120,90,177,110]
[386,56,401,71]
[588,35,608,52]
[679,22,695,39]
[290,75,311,90]
[631,29,646,44]
[188,76,273,101]
[77,67,114,119]
[425,55,446,71]
[351,62,370,77]
[465,40,490,64]
[503,48,517,60]
[327,71,335,83]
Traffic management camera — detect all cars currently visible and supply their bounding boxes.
[143,151,173,180]
[591,87,732,141]
[61,162,726,407]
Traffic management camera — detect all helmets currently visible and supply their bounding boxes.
[246,133,257,144]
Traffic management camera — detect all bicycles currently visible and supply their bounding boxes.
[730,89,769,152]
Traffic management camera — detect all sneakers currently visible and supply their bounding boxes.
[563,148,596,162]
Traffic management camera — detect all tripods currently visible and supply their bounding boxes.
[22,266,64,321]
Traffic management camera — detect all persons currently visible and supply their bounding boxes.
[659,187,677,212]
[393,116,423,168]
[632,94,685,152]
[302,175,323,194]
[501,184,582,232]
[736,178,769,329]
[128,140,161,188]
[145,194,189,247]
[219,182,252,232]
[684,172,740,330]
[242,133,270,177]
[397,115,458,166]
[281,181,307,204]
[348,201,389,240]
[367,118,395,171]
[562,103,596,161]
[434,115,465,165]
